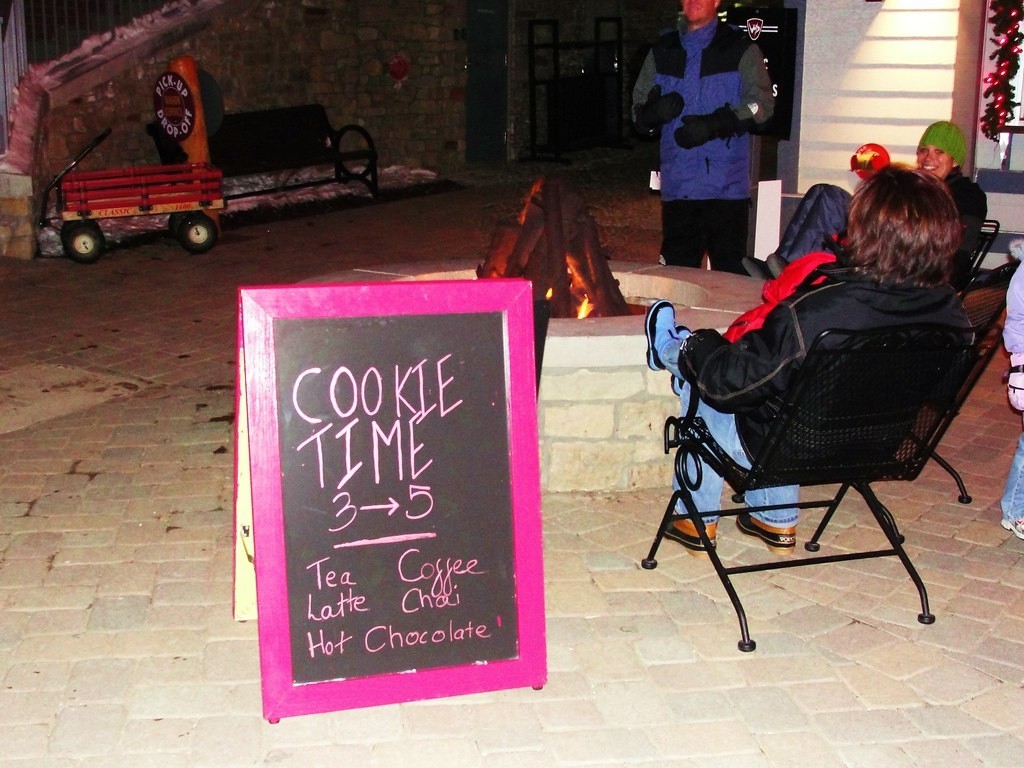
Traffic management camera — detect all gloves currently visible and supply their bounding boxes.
[634,84,685,134]
[1007,354,1024,410]
[674,102,747,149]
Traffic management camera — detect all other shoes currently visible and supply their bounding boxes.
[741,256,775,279]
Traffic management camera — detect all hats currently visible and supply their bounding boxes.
[919,121,966,166]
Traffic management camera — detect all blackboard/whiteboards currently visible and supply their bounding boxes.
[228,277,551,727]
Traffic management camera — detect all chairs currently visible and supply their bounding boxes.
[640,323,986,653]
[850,219,1021,503]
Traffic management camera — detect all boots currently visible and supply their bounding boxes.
[671,325,691,396]
[646,299,685,382]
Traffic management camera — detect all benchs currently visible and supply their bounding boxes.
[144,102,387,227]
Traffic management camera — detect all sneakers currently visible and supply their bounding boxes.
[664,519,716,557]
[736,513,796,555]
[1001,518,1024,539]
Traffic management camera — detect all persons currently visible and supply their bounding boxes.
[632,0,773,277]
[744,121,987,284]
[1000,239,1024,539]
[647,166,964,560]
